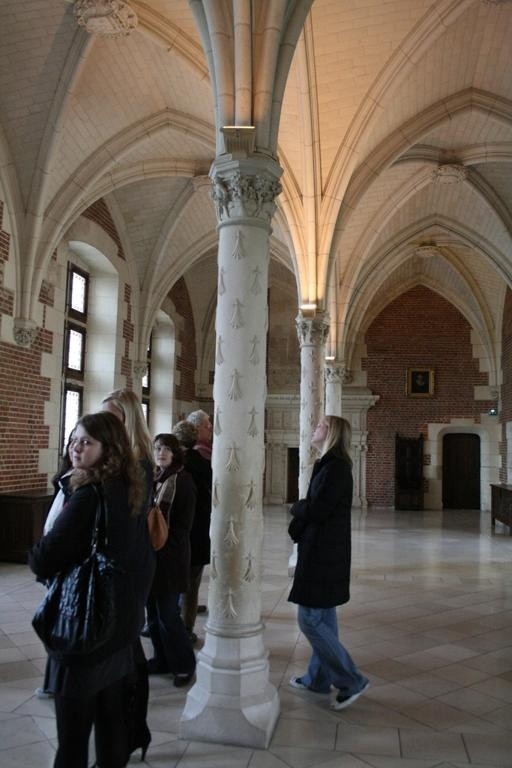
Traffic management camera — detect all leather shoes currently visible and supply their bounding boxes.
[172,673,192,688]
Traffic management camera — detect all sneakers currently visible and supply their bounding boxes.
[289,675,307,689]
[35,687,55,699]
[330,680,369,711]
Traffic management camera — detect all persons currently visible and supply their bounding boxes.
[186,408,213,615]
[41,427,76,538]
[138,420,213,647]
[27,411,157,768]
[287,415,372,710]
[98,387,158,764]
[144,433,197,688]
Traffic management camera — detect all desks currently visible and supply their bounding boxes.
[489,482,511,534]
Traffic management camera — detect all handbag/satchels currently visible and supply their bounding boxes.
[31,553,117,657]
[147,506,169,552]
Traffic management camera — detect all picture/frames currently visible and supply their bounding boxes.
[405,367,435,398]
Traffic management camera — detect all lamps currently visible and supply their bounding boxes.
[219,123,257,153]
[325,356,336,364]
[299,302,317,316]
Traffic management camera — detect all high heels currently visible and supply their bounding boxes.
[130,725,152,762]
